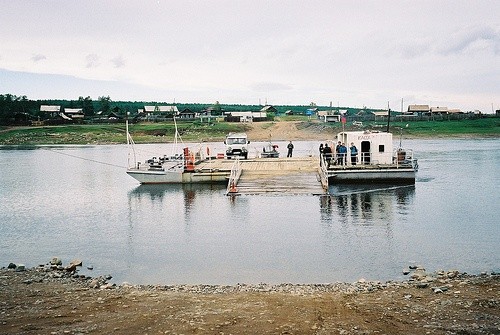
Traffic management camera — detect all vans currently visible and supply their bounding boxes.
[224,132,250,159]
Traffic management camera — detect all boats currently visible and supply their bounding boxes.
[125,131,418,195]
[261,133,279,158]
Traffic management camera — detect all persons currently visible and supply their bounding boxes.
[286,141,294,157]
[350,142,358,166]
[319,141,348,166]
